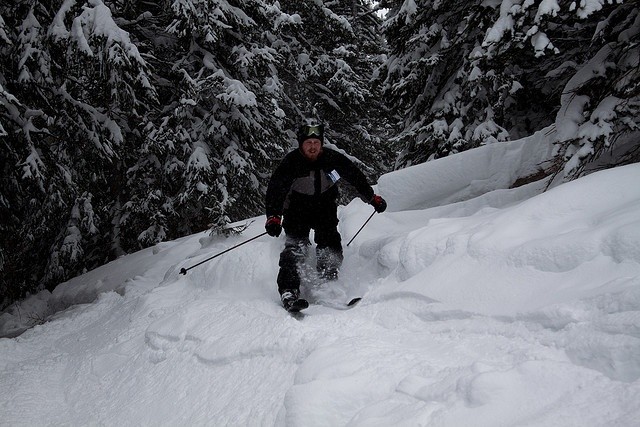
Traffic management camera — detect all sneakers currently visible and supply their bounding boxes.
[280,289,298,311]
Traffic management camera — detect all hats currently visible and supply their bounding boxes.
[299,124,324,147]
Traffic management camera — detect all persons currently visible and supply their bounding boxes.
[264,123,388,312]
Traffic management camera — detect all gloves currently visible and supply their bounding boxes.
[367,194,387,213]
[265,207,283,237]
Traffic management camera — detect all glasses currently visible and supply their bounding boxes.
[303,124,322,136]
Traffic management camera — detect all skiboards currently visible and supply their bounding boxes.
[284,289,364,311]
